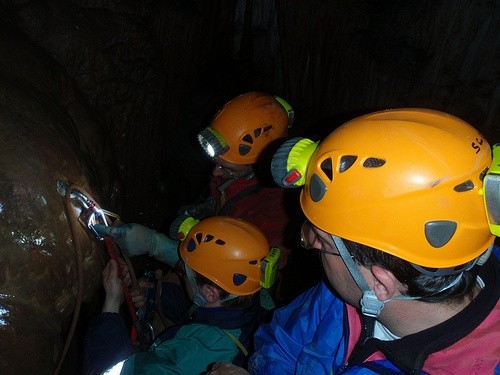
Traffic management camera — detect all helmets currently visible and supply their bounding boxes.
[169,214,281,296]
[271,107,499,277]
[197,91,295,165]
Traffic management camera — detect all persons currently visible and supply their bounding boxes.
[93,91,299,303]
[205,107,500,375]
[82,214,281,375]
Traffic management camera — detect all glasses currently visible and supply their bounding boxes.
[300,220,400,278]
[219,165,249,179]
